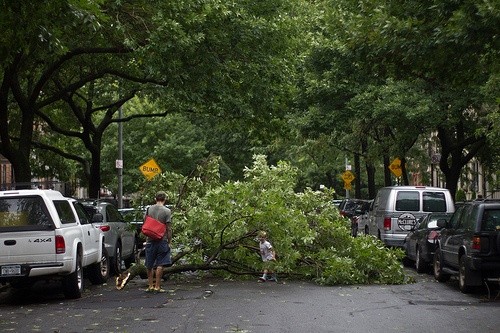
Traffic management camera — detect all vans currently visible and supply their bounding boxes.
[365,186,456,247]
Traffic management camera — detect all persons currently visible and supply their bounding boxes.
[353,213,359,238]
[258,230,277,282]
[141,191,172,293]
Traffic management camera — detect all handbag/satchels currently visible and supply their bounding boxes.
[141,207,167,241]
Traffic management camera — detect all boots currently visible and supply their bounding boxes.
[260,274,267,281]
[268,274,276,281]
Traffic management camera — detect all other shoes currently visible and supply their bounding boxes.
[144,287,153,292]
[154,287,165,293]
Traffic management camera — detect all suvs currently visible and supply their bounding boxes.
[433,201,500,293]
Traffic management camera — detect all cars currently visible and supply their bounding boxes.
[117,209,146,256]
[402,213,453,273]
[339,199,370,218]
[78,202,139,274]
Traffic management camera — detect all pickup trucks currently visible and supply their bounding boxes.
[0,189,111,298]
